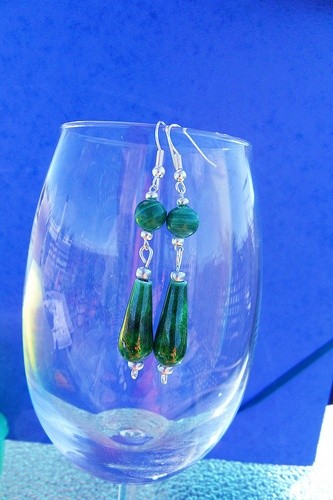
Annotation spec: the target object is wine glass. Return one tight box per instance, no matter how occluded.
[23,121,261,499]
